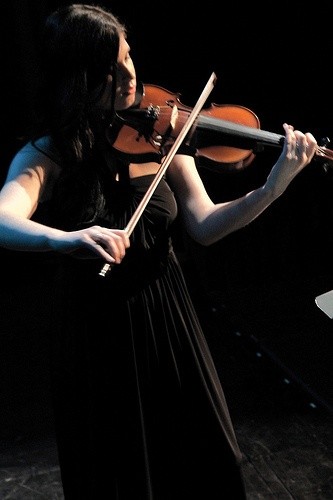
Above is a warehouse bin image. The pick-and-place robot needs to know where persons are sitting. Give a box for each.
[0,5,318,499]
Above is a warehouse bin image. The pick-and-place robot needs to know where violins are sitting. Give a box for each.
[104,82,333,170]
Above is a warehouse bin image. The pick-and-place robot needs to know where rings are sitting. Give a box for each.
[295,142,305,153]
